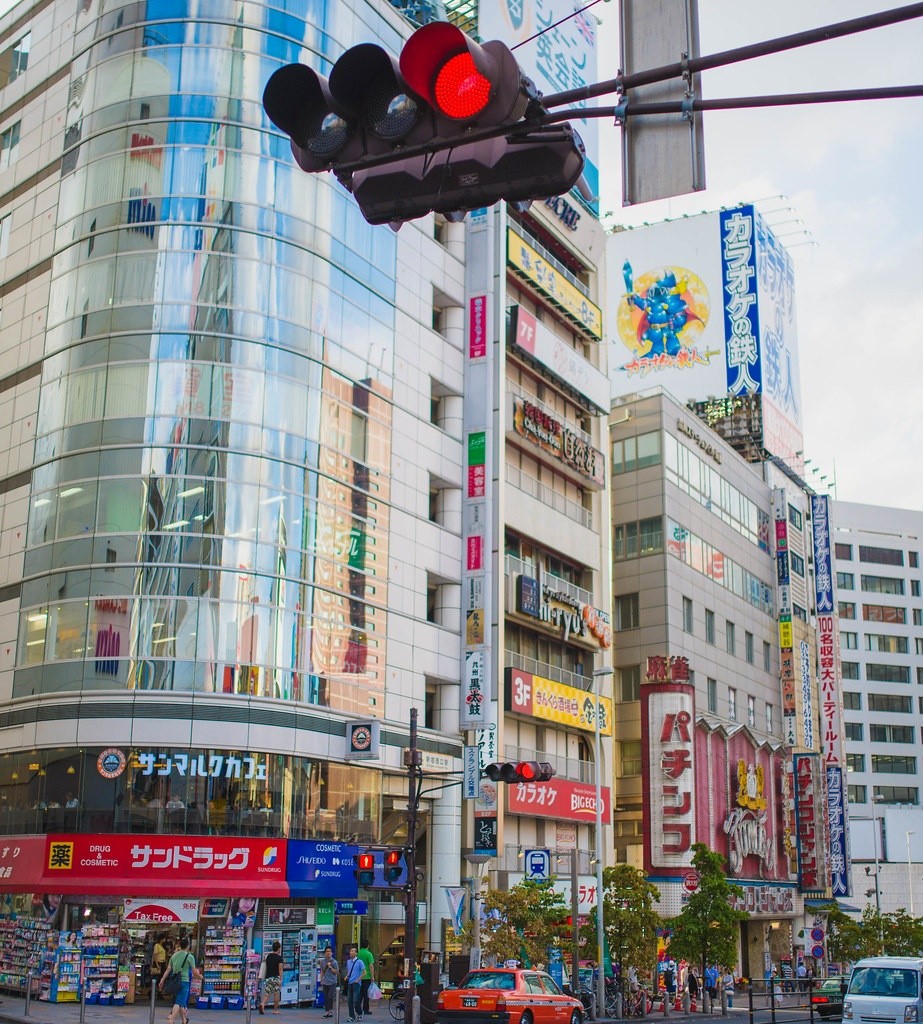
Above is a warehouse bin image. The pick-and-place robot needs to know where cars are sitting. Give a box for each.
[811,974,851,1020]
[436,965,587,1024]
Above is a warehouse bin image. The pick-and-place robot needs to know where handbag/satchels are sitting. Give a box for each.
[343,982,349,996]
[258,953,269,980]
[367,982,382,999]
[726,990,734,995]
[164,972,182,993]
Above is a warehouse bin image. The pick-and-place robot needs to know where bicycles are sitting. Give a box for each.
[388,987,406,1022]
[556,968,654,1021]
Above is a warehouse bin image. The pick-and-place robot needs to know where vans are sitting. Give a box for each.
[841,955,923,1024]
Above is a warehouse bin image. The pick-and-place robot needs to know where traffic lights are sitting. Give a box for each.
[357,852,376,886]
[263,21,535,175]
[485,760,543,784]
[383,849,403,882]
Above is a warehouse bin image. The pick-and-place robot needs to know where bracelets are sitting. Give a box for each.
[329,967,333,970]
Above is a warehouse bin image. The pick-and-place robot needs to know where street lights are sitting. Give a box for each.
[592,668,615,1019]
[871,795,885,955]
[906,831,917,927]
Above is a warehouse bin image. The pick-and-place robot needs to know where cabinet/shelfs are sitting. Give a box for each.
[130,929,174,994]
[203,925,246,995]
[0,921,119,1002]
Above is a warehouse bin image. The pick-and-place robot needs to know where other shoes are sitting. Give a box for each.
[259,1004,264,1015]
[358,1015,364,1020]
[273,1010,280,1014]
[182,1018,189,1024]
[346,1018,355,1022]
[166,1015,172,1019]
[364,1011,372,1014]
[321,1013,333,1018]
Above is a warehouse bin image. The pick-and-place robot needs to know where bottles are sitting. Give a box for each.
[57,944,119,993]
[203,925,246,990]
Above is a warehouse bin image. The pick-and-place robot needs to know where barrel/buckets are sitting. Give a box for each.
[195,995,243,1009]
[85,994,124,1005]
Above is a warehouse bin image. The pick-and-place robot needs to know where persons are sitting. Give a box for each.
[706,963,718,999]
[722,968,734,1007]
[259,941,283,1013]
[772,971,783,1007]
[357,940,374,1014]
[807,965,815,991]
[230,898,256,927]
[67,932,78,949]
[148,934,165,1000]
[686,966,699,999]
[794,962,807,996]
[320,946,338,1017]
[665,960,678,1005]
[783,967,795,996]
[344,948,366,1021]
[159,939,203,1024]
